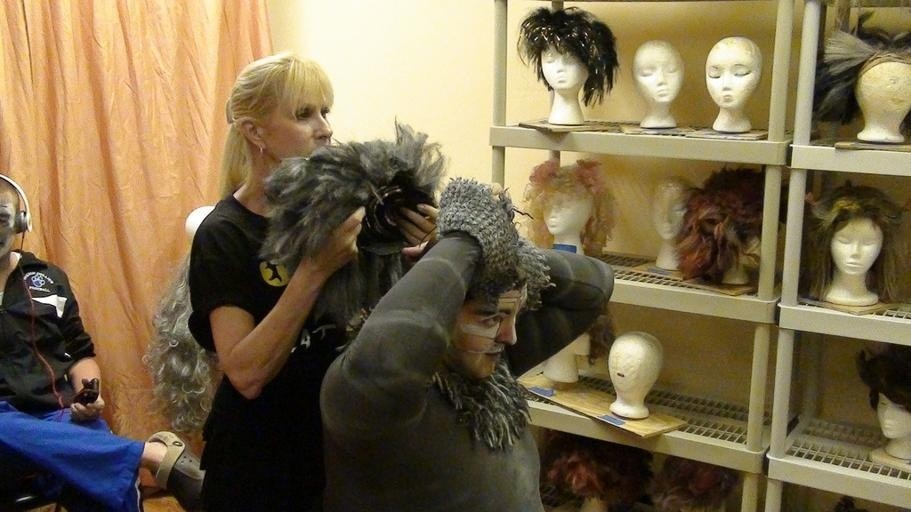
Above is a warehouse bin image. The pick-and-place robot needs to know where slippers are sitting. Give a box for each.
[146,432,206,512]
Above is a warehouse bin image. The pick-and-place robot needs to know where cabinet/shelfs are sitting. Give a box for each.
[488,1,798,510]
[763,1,911,510]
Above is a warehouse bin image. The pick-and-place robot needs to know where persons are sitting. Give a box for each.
[815,25,909,143]
[856,345,911,459]
[676,167,788,282]
[533,430,737,512]
[607,331,664,419]
[806,185,909,307]
[190,56,441,509]
[143,199,227,441]
[631,40,685,128]
[0,174,212,511]
[517,8,618,125]
[522,160,615,257]
[705,37,762,133]
[319,178,616,510]
[542,306,614,382]
[652,177,695,270]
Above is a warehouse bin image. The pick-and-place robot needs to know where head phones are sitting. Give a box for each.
[0,173,32,235]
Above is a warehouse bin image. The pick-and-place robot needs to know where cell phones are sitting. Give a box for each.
[73,379,99,405]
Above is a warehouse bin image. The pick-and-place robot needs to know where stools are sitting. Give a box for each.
[0,472,66,512]
